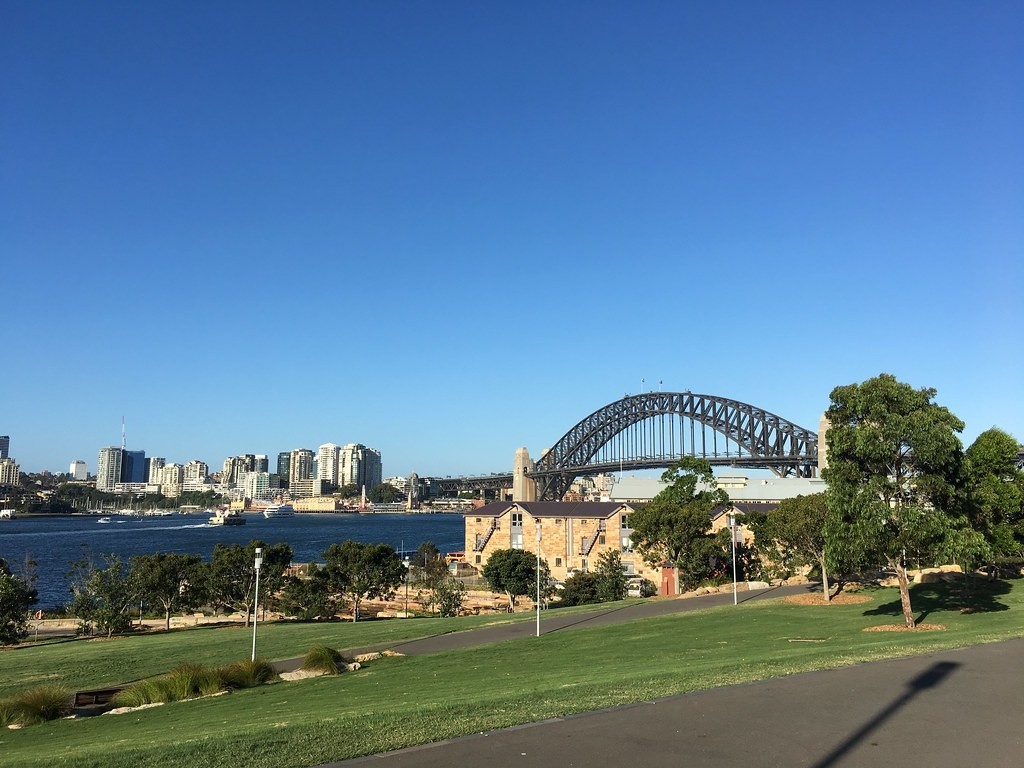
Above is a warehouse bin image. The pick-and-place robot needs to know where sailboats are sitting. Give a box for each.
[82,496,220,517]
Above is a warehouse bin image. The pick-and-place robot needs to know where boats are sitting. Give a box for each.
[262,503,295,518]
[208,505,247,526]
[95,517,113,524]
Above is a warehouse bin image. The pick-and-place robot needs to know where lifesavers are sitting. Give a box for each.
[35,611,45,620]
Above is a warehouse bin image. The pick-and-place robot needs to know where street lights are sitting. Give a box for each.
[251,547,265,664]
[729,512,738,606]
[534,525,544,638]
